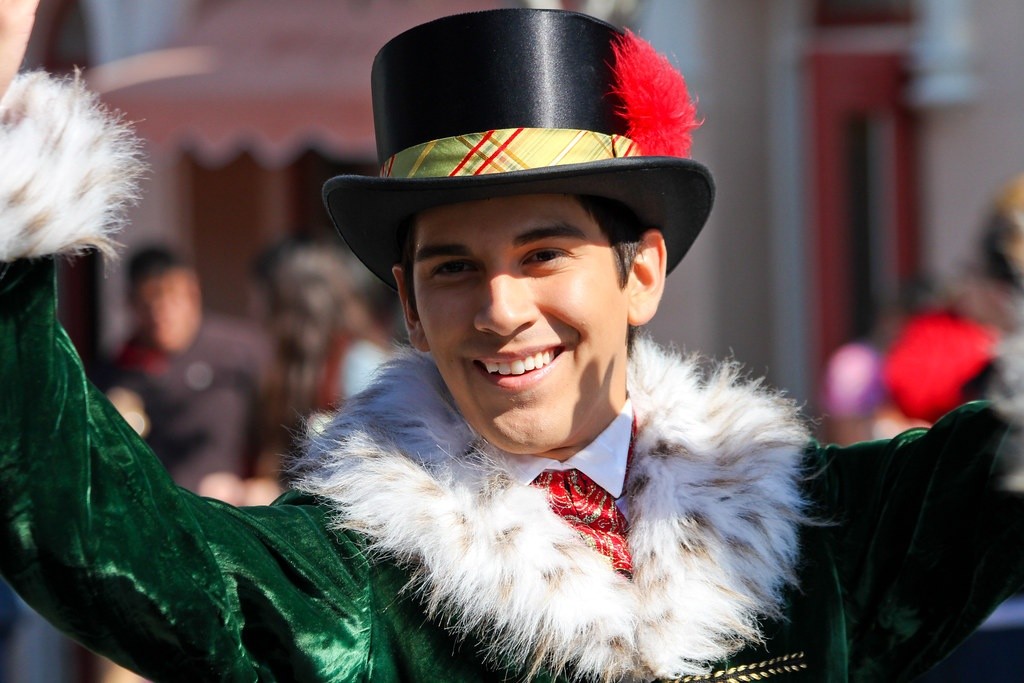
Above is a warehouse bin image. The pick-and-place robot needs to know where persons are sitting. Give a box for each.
[823,171,1024,447]
[104,238,402,507]
[0,0,1024,683]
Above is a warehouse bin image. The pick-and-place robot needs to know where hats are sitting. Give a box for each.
[322,8,717,293]
